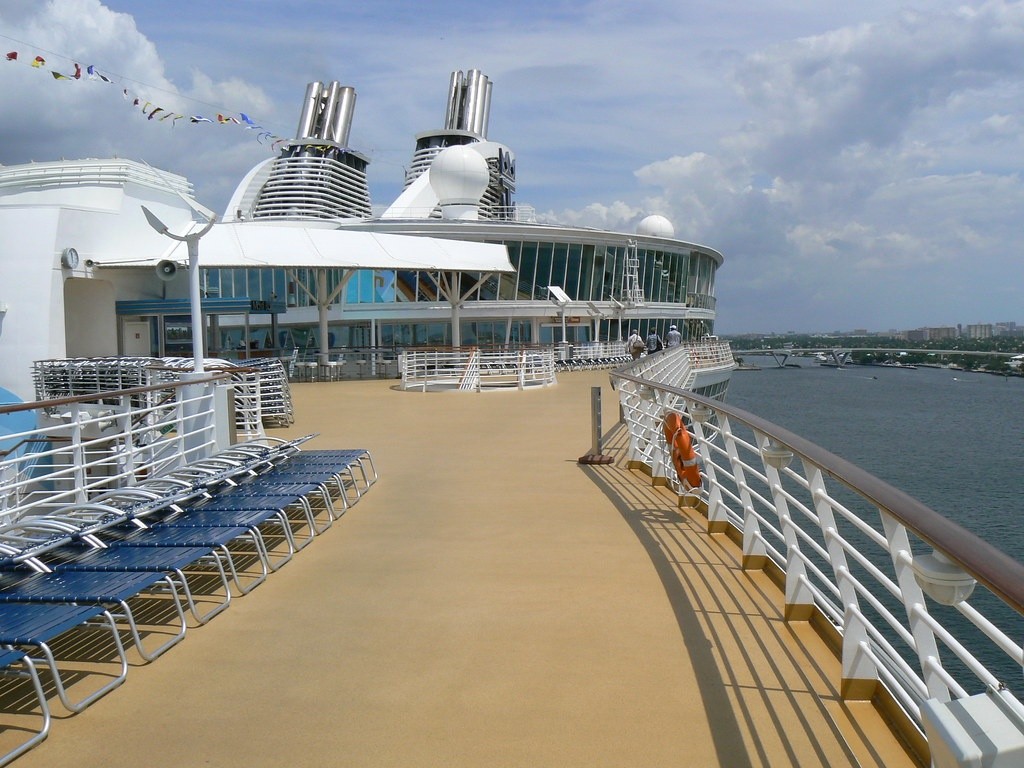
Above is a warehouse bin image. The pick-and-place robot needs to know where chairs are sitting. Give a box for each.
[0,432,378,768]
[441,356,634,377]
[29,354,294,429]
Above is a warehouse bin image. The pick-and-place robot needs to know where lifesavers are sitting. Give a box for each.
[666,412,705,491]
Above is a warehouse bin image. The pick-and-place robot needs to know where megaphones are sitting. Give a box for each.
[156,260,178,281]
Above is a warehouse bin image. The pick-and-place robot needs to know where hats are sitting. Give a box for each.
[651,327,656,331]
[670,325,676,329]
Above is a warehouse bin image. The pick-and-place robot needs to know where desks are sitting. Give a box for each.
[357,360,391,379]
[295,362,317,382]
[320,360,346,383]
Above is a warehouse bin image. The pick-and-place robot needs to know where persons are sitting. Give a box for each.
[666,325,682,348]
[646,327,663,354]
[625,329,643,360]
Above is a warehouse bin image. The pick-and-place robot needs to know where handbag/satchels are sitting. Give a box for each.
[633,335,643,348]
[657,336,662,351]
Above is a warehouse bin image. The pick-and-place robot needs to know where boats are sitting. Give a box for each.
[892,362,917,369]
[813,353,828,363]
[733,362,762,370]
